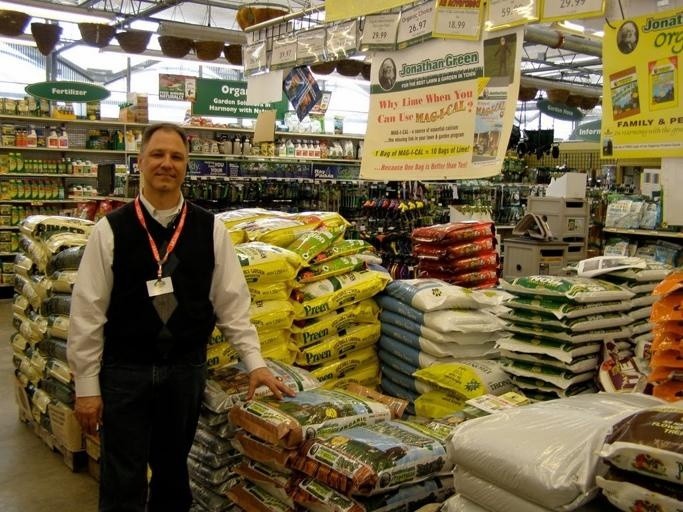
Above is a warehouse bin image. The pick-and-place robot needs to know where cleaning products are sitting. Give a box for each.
[276,138,321,158]
[26,124,38,148]
[59,125,69,149]
[46,125,60,148]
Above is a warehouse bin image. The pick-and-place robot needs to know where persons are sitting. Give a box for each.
[64,121,299,511]
[379,58,394,90]
[493,38,512,75]
[618,22,637,55]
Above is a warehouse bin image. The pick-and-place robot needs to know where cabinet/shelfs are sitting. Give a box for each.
[0,114,364,288]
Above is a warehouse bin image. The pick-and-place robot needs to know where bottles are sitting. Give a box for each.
[209,134,363,159]
[126,129,138,152]
[0,96,104,251]
